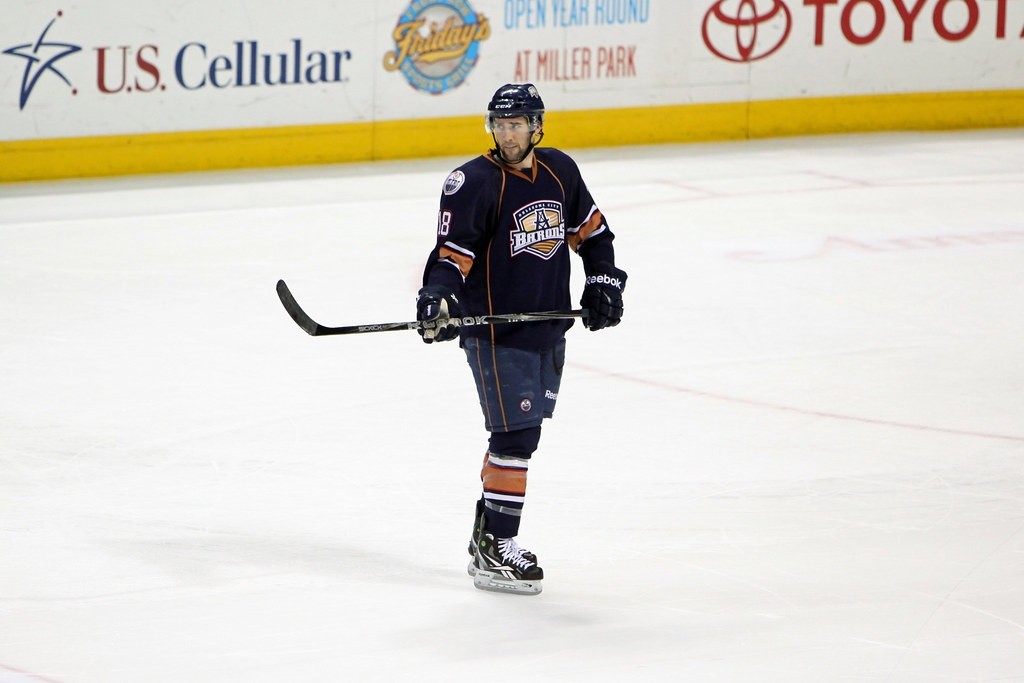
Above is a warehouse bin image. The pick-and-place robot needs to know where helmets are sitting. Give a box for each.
[485,84,546,134]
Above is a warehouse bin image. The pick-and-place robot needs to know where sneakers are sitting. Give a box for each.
[468,497,544,596]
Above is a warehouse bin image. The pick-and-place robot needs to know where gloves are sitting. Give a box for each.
[580,264,628,332]
[416,285,462,343]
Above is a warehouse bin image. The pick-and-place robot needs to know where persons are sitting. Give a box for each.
[416,83,628,595]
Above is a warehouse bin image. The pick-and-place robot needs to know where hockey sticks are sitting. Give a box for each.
[276,280,590,339]
